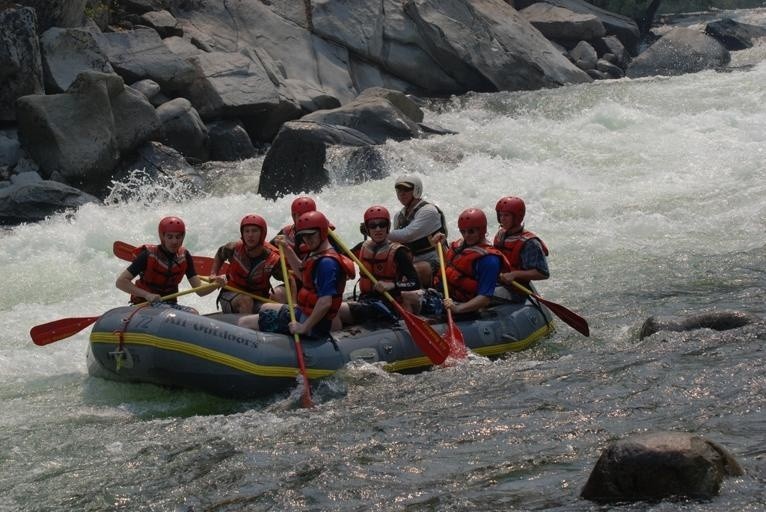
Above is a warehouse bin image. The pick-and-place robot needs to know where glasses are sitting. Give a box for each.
[460,229,476,235]
[368,221,387,228]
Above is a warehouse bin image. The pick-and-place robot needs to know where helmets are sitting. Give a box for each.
[496,197,526,225]
[295,211,329,241]
[158,216,185,248]
[395,173,422,200]
[364,206,390,234]
[292,197,316,224]
[241,215,267,246]
[458,209,487,238]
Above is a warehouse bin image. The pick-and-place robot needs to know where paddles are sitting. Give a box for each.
[30,280,223,347]
[112,240,278,304]
[278,236,312,409]
[498,273,590,338]
[191,255,231,283]
[437,239,465,360]
[327,226,450,365]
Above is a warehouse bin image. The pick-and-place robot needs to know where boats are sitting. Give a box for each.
[87,294,552,389]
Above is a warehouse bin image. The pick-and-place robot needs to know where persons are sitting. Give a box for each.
[115,217,227,316]
[362,173,447,284]
[269,197,335,280]
[495,195,550,287]
[403,208,500,321]
[210,215,282,315]
[332,207,418,330]
[237,210,355,341]
